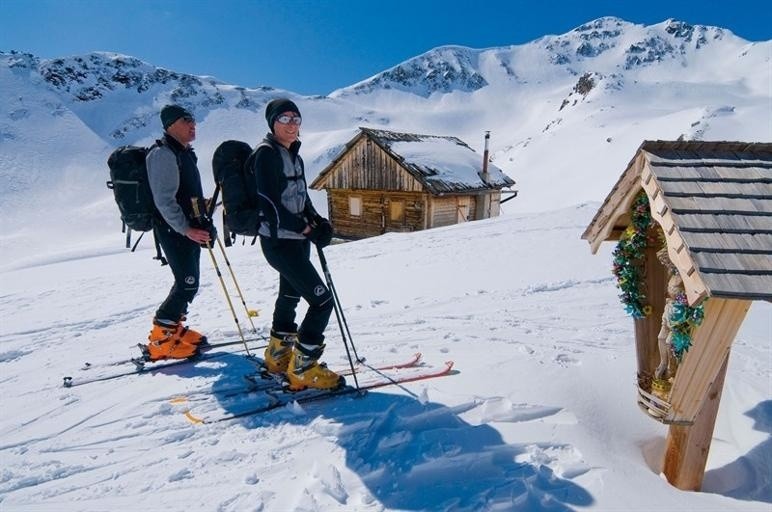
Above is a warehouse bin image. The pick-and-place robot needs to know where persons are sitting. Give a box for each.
[255,98,345,391]
[143,104,216,359]
[651,272,685,380]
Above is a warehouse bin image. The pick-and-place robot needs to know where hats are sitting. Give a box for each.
[266,99,302,135]
[161,105,185,129]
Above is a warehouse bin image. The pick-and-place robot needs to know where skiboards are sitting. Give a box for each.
[64,337,271,388]
[168,353,453,423]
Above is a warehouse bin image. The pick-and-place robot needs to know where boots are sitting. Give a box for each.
[287,339,345,389]
[174,322,206,345]
[149,316,199,359]
[264,329,296,373]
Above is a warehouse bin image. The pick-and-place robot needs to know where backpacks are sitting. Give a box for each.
[211,140,283,247]
[107,139,185,267]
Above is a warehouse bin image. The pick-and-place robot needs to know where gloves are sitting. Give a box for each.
[303,218,334,247]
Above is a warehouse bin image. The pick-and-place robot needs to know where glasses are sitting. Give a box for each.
[183,114,196,123]
[277,115,302,125]
